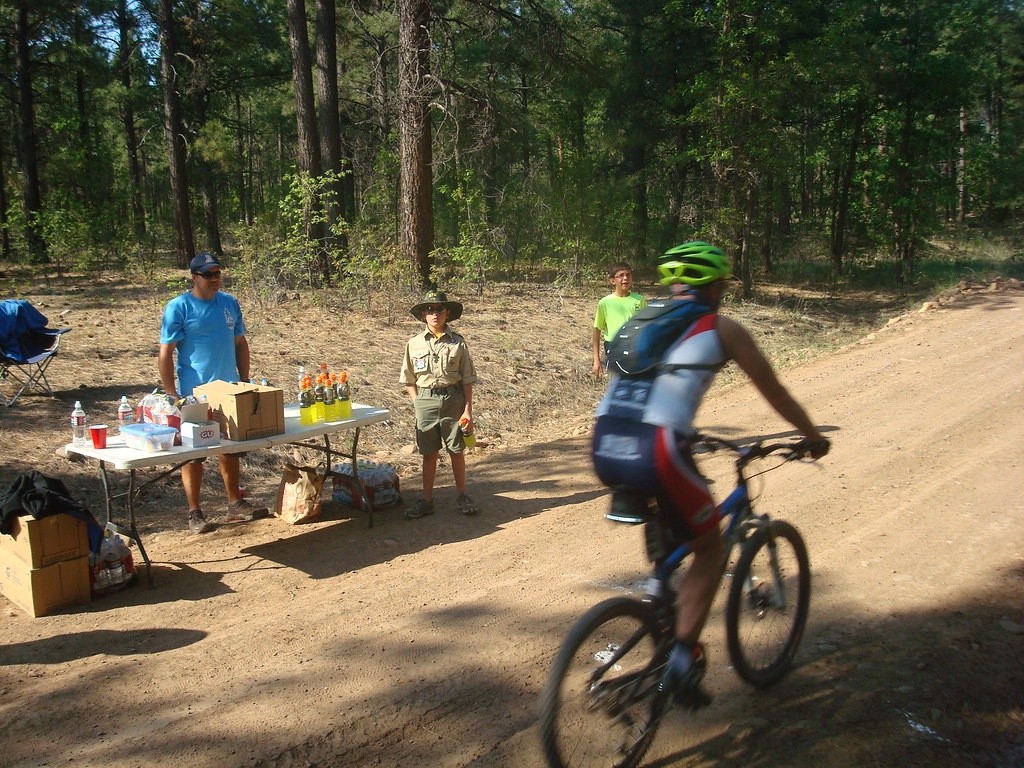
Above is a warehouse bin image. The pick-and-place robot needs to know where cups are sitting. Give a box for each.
[89,425,108,449]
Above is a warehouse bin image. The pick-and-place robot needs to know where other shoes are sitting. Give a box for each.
[404,499,436,518]
[661,642,715,711]
[456,493,479,514]
[639,590,680,613]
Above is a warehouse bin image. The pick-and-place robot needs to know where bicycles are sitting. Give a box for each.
[538,434,813,768]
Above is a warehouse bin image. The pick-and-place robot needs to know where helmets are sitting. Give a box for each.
[657,240,733,294]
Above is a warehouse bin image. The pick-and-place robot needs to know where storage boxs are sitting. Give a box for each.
[0,510,90,569]
[0,545,91,618]
[180,403,220,448]
[117,423,179,452]
[192,379,285,441]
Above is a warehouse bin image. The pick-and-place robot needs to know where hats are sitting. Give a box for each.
[409,289,463,322]
[190,253,222,274]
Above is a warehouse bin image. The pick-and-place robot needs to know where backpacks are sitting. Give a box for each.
[607,299,723,378]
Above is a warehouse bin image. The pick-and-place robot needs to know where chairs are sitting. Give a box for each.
[0,299,72,406]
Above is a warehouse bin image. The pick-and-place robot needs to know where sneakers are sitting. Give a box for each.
[188,510,209,535]
[226,500,269,522]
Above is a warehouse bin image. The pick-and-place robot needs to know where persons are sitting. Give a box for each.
[592,242,831,708]
[158,252,269,535]
[398,289,479,519]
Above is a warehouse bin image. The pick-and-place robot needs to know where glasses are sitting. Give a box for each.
[195,271,222,279]
[425,305,446,313]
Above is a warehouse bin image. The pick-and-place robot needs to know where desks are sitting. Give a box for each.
[64,401,390,595]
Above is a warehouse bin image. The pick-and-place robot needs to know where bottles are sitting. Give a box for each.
[262,378,267,385]
[460,417,476,447]
[88,529,137,590]
[299,366,305,390]
[314,372,336,423]
[250,379,255,384]
[229,380,233,383]
[200,395,212,421]
[329,372,352,421]
[333,459,399,510]
[319,362,329,380]
[71,401,86,448]
[118,396,134,440]
[298,375,317,426]
